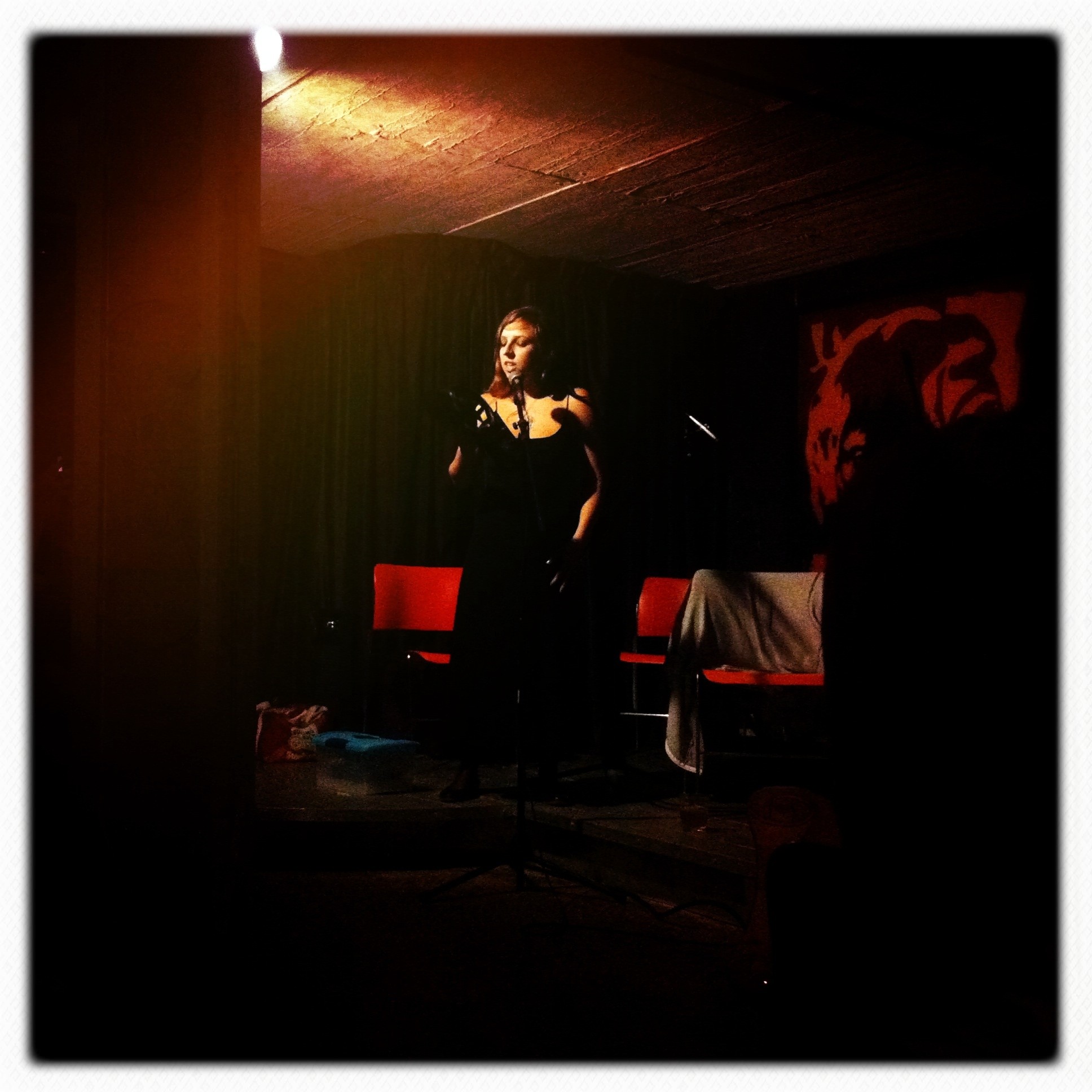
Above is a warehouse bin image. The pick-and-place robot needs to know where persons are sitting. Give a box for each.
[445,307,620,798]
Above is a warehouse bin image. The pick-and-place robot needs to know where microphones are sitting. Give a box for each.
[513,377,525,405]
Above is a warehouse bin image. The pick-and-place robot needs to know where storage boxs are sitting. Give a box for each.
[312,729,421,795]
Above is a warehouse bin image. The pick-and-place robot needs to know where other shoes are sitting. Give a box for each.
[439,768,479,802]
[541,765,565,805]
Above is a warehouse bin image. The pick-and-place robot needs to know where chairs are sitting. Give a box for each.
[352,566,835,765]
